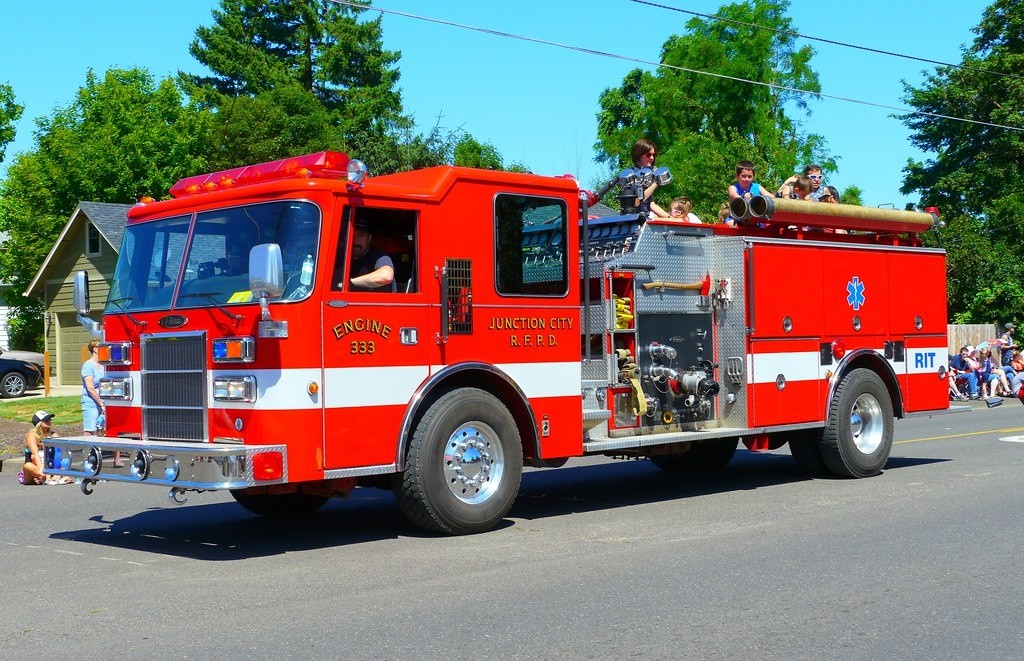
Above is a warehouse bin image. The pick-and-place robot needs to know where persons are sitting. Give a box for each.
[337,218,397,293]
[619,139,675,219]
[674,195,703,224]
[80,339,125,472]
[655,201,690,222]
[775,164,854,235]
[18,410,73,484]
[724,161,775,228]
[999,323,1019,367]
[948,347,1024,402]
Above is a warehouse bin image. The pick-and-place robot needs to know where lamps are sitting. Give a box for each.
[45,310,51,325]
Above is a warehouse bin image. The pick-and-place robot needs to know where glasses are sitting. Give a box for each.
[645,153,656,158]
[806,173,822,180]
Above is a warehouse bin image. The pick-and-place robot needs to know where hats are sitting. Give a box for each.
[32,410,55,426]
[948,353,954,361]
[1005,323,1016,329]
[344,217,377,234]
[967,345,979,356]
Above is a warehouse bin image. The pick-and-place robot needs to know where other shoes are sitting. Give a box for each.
[970,395,979,399]
[997,391,1013,397]
[114,461,125,468]
[957,394,968,401]
[979,396,990,401]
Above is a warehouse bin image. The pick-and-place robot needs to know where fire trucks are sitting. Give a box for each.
[41,149,947,537]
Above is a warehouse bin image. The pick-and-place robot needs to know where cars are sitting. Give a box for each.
[0,344,44,398]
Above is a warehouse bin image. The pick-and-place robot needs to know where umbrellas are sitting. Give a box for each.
[975,339,1008,351]
